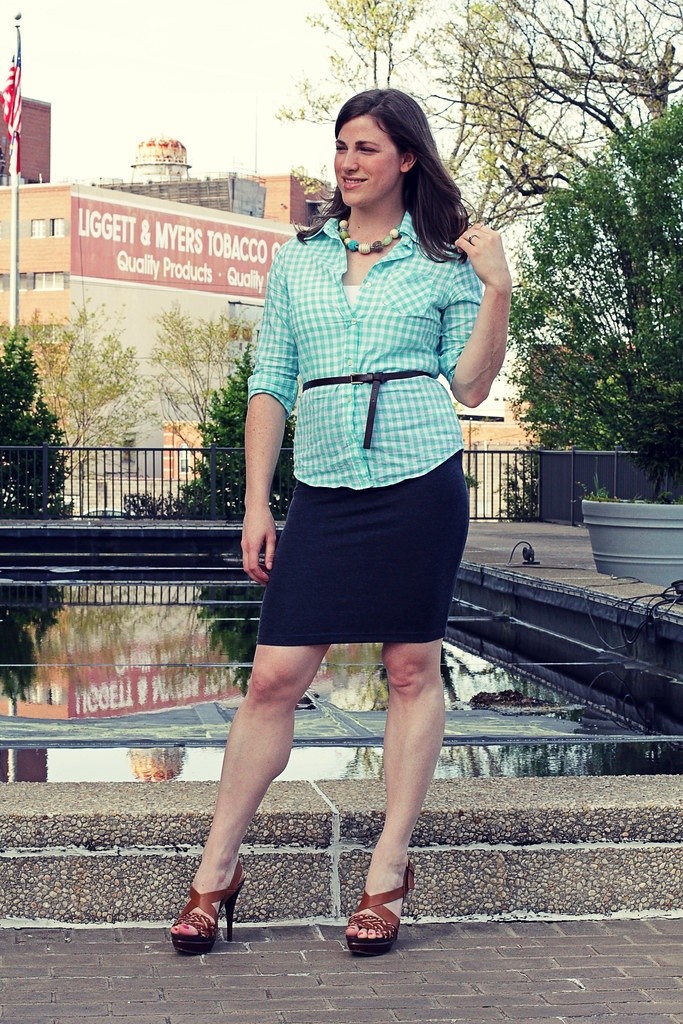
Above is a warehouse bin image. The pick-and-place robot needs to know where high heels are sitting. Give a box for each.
[171,859,246,953]
[346,859,415,955]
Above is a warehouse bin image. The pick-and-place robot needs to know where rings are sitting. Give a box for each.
[469,234,478,245]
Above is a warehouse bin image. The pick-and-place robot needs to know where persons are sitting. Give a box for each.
[172,89,511,955]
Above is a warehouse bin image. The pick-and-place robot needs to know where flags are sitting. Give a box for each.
[0,39,22,173]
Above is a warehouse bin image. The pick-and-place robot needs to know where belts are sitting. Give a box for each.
[303,370,432,449]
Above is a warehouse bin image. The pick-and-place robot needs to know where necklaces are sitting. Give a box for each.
[339,220,400,254]
